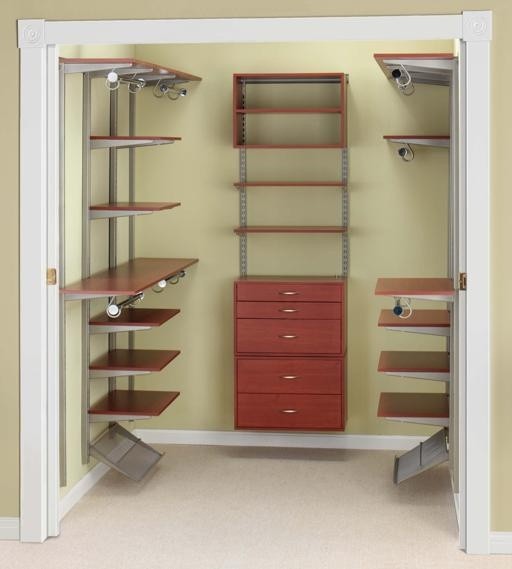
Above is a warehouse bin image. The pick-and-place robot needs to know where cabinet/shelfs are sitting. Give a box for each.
[230,73,350,277]
[58,56,202,487]
[232,280,348,432]
[372,51,458,486]
